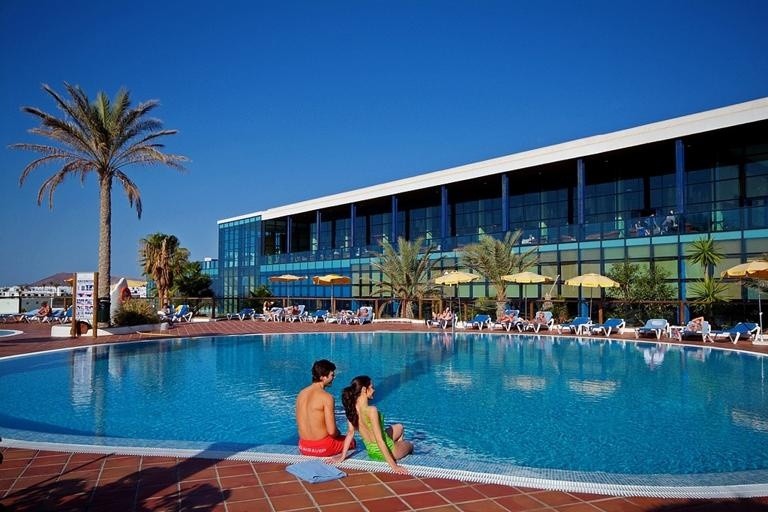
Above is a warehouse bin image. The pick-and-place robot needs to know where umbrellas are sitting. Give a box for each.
[437,362,477,394]
[500,366,548,395]
[269,273,309,304]
[498,270,554,317]
[564,368,622,400]
[310,272,351,306]
[717,258,768,335]
[564,271,621,319]
[433,272,481,317]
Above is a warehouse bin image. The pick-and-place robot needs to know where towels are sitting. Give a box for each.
[285,457,346,485]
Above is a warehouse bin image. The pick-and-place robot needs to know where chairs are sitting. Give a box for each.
[0,304,75,324]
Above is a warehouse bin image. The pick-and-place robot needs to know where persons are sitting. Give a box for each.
[326,373,417,478]
[634,209,679,236]
[292,358,358,457]
[529,313,547,325]
[262,300,370,324]
[500,312,516,322]
[688,315,704,333]
[159,302,177,327]
[432,306,454,321]
[35,299,52,323]
